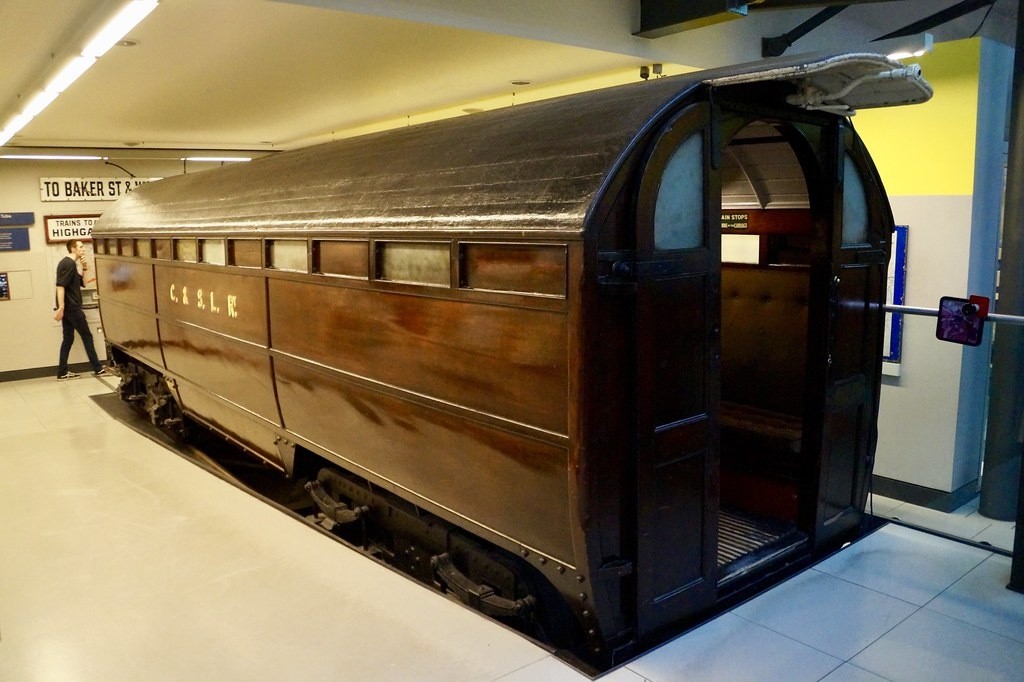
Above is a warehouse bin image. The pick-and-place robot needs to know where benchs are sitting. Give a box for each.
[716,397,804,450]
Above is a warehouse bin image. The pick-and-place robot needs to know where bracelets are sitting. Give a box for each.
[83,268,88,271]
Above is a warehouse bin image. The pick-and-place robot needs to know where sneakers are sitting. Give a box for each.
[57,371,81,380]
[95,366,116,377]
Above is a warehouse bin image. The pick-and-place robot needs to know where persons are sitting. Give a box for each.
[52,239,114,381]
